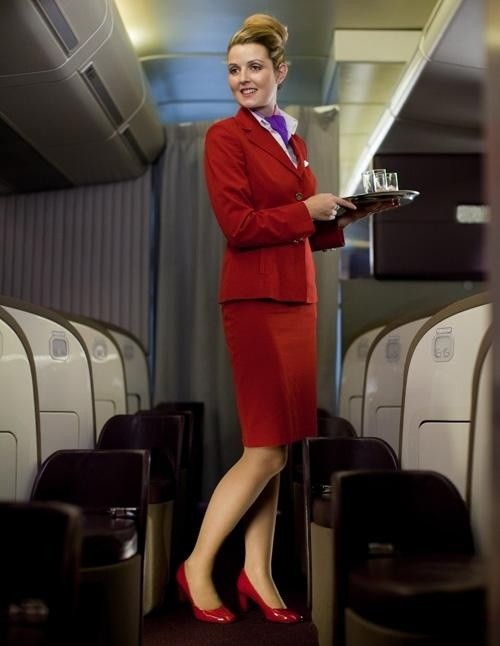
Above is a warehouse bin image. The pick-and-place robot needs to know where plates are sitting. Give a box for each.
[339,190,420,208]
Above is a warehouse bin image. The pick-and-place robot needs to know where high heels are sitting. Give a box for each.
[176,561,236,623]
[238,569,305,624]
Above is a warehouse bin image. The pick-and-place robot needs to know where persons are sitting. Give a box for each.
[175,14,399,624]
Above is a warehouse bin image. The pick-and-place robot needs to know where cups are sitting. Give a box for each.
[362,169,398,193]
